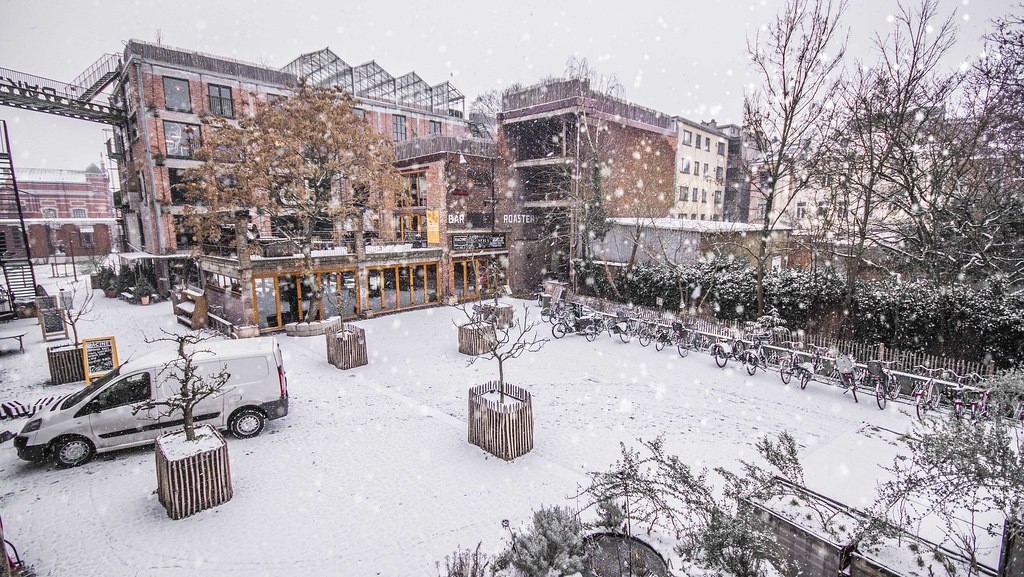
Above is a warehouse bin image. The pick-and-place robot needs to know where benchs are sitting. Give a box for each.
[121,292,134,304]
[0,330,29,354]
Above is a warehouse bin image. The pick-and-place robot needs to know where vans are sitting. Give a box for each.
[13,333,289,469]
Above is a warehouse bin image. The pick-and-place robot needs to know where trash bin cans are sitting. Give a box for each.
[541,294,551,315]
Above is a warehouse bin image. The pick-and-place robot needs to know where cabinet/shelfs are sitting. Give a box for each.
[175,285,208,331]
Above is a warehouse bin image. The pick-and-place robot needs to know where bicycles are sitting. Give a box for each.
[742,333,768,376]
[534,285,710,358]
[944,368,996,422]
[868,360,903,411]
[800,344,830,390]
[713,327,746,368]
[912,365,946,421]
[834,354,859,402]
[778,341,801,379]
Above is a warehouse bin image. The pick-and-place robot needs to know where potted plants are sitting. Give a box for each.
[106,279,119,298]
[140,289,150,305]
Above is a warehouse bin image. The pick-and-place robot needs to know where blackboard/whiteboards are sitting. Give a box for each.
[34,296,58,317]
[36,306,68,337]
[82,337,118,379]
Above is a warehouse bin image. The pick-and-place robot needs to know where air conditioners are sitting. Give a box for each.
[114,190,129,209]
[105,137,123,157]
